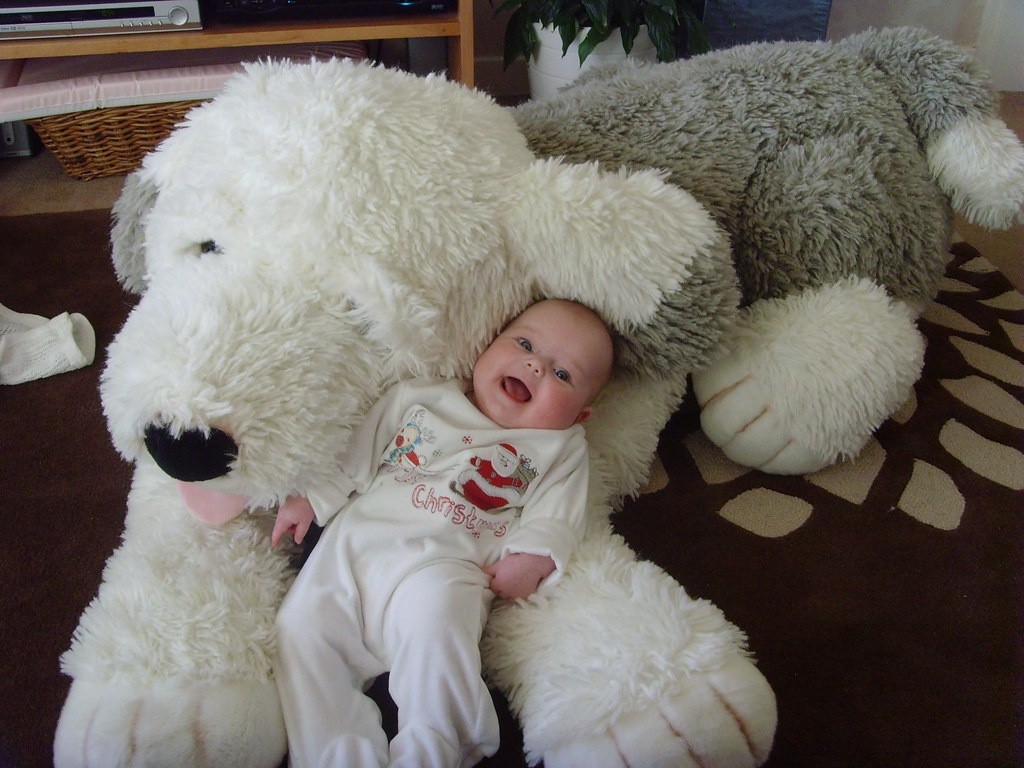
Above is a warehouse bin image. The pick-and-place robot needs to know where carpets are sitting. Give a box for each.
[0,207,1024,768]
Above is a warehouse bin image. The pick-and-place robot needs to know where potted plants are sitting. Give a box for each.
[493,0,737,102]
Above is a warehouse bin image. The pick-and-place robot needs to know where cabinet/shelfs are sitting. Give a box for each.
[0,0,475,216]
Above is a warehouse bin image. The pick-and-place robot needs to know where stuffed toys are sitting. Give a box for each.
[54,26,1024,768]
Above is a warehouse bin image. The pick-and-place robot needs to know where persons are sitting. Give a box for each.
[270,301,614,768]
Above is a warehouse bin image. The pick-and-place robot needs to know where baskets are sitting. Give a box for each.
[22,98,214,183]
